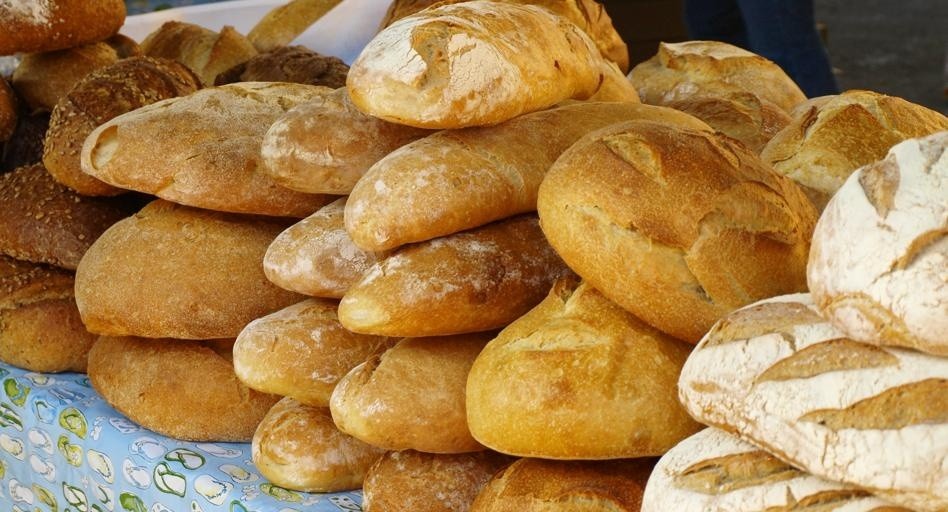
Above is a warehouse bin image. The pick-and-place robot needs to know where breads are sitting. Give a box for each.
[198,24,258,88]
[12,43,118,109]
[371,2,629,74]
[264,196,397,301]
[475,458,644,512]
[217,46,350,87]
[2,259,87,375]
[80,80,341,226]
[246,0,344,47]
[81,337,278,441]
[538,117,821,345]
[767,91,948,199]
[809,129,948,356]
[341,101,719,255]
[258,84,430,197]
[333,337,504,456]
[362,452,497,511]
[41,53,198,199]
[345,1,606,131]
[470,286,697,460]
[678,287,948,511]
[142,21,218,74]
[334,213,565,338]
[638,427,898,510]
[243,396,380,493]
[2,0,129,57]
[234,300,404,401]
[0,164,129,272]
[72,199,303,341]
[629,36,808,174]
